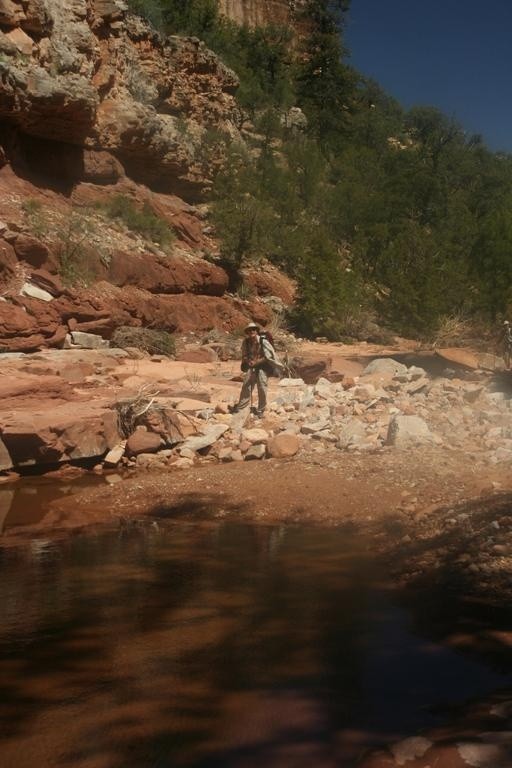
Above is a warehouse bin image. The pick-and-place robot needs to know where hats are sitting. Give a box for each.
[244,322,260,334]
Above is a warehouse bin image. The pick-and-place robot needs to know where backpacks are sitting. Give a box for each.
[259,331,276,353]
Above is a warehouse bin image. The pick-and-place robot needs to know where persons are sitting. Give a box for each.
[227,322,283,415]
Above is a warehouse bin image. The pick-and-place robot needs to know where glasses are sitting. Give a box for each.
[248,329,256,332]
